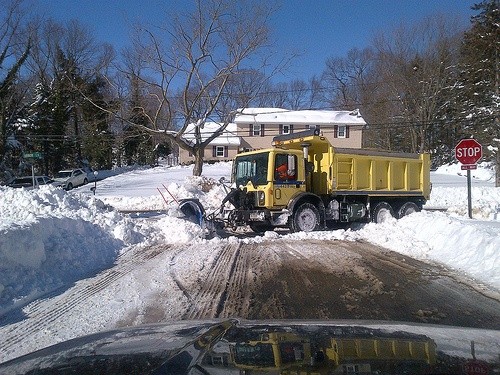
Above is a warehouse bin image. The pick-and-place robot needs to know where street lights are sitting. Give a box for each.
[92,171,97,190]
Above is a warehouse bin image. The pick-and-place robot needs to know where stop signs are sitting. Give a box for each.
[455,139,481,165]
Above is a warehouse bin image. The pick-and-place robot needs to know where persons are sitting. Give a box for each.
[274,158,294,180]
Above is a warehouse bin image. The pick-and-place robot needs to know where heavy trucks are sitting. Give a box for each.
[214,129,431,235]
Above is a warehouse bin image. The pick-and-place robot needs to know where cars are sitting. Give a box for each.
[52,168,88,191]
[9,175,62,193]
